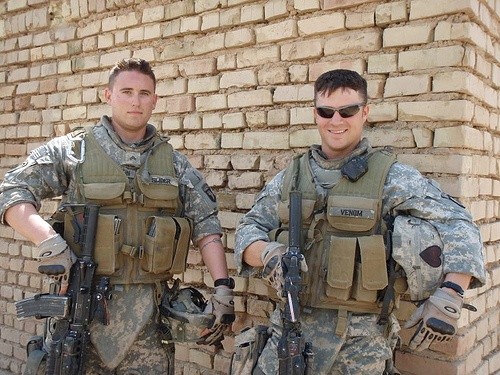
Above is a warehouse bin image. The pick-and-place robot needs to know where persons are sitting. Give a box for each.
[0,58,235,375]
[234,69,486,375]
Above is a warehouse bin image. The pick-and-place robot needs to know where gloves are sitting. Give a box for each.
[194,286,239,346]
[402,288,463,351]
[260,242,309,293]
[31,235,77,297]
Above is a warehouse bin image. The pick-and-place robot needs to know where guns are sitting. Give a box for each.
[274,192,316,375]
[15,203,100,375]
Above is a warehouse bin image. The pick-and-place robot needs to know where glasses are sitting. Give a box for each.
[315,101,367,119]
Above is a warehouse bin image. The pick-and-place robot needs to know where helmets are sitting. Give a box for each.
[393,216,445,302]
[161,288,207,344]
